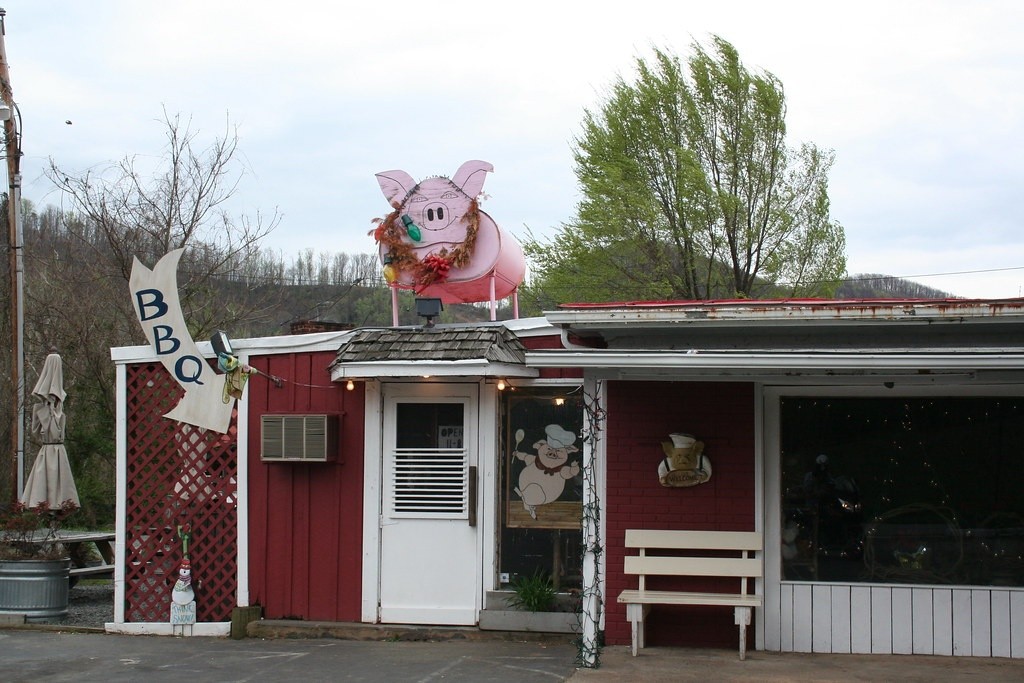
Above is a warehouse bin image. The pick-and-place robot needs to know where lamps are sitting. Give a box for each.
[347,377,354,390]
[415,298,444,328]
[210,330,233,357]
[498,376,506,390]
[552,394,566,405]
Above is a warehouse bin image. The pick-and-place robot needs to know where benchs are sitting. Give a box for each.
[69,562,151,576]
[618,529,763,661]
[132,546,170,556]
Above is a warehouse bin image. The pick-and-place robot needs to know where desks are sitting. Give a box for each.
[0,527,116,566]
[137,527,172,561]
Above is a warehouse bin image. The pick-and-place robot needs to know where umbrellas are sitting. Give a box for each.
[20,346,82,556]
[174,422,210,512]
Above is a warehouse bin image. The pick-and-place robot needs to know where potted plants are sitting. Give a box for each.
[0,500,85,624]
